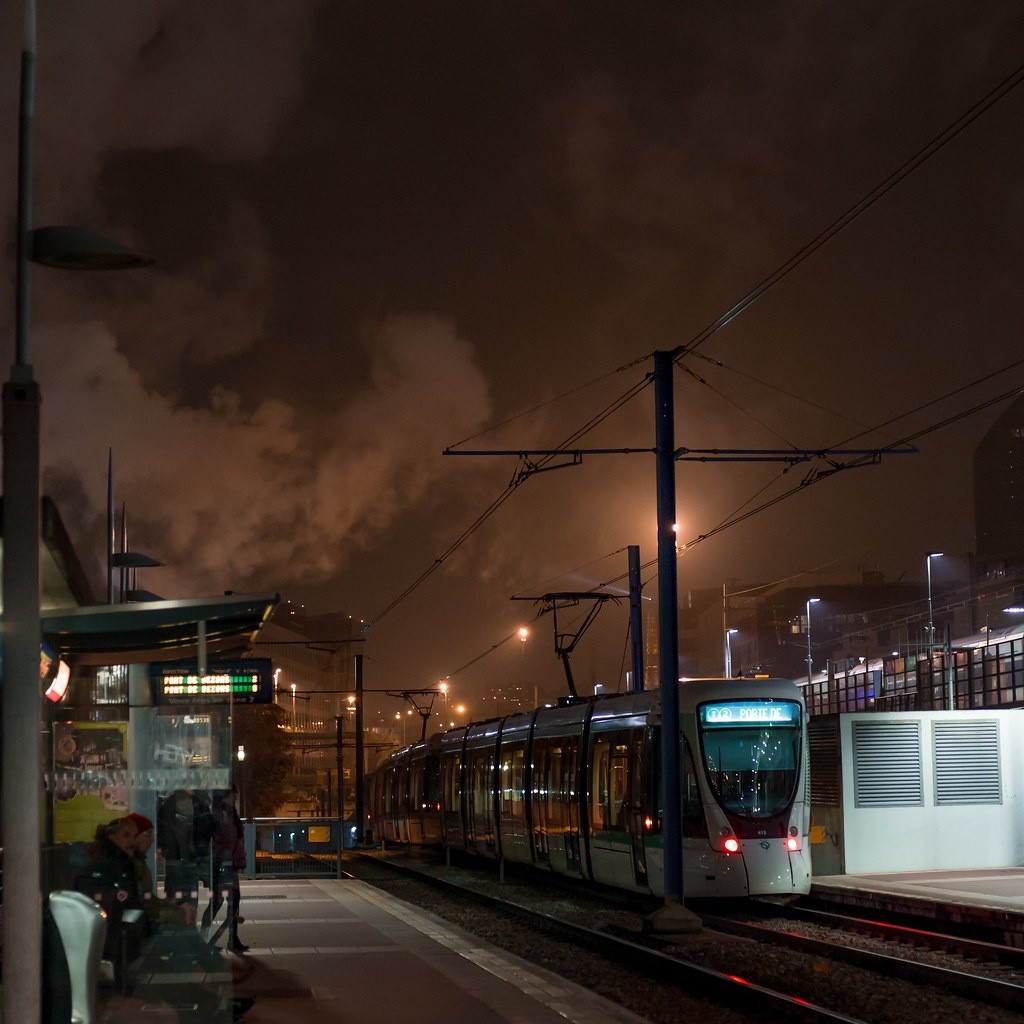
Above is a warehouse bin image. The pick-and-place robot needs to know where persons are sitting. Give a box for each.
[157,765,250,953]
[68,813,257,1024]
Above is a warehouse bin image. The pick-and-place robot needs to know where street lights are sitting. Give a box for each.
[806,599,821,682]
[928,553,944,651]
[726,629,739,679]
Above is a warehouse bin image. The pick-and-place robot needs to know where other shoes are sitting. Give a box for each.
[227,935,248,951]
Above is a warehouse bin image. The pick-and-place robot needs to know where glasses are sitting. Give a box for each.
[142,831,152,837]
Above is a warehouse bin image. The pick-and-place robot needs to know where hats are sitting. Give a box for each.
[128,813,154,839]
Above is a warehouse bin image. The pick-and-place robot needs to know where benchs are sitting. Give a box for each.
[68,879,145,994]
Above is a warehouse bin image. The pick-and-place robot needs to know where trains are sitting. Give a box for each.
[365,591,809,909]
